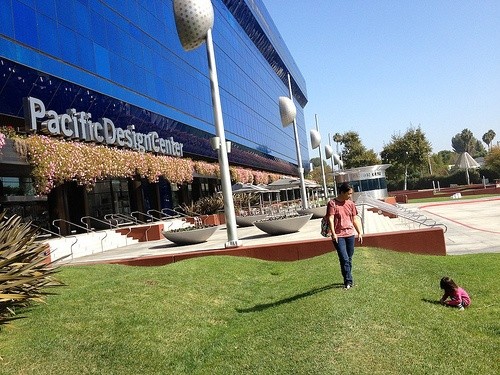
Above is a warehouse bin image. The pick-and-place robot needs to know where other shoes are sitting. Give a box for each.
[343,284,350,290]
[456,303,464,311]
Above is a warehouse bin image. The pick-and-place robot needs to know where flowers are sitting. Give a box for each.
[0,124,299,199]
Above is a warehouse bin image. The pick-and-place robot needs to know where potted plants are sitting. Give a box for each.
[162,224,218,244]
[253,212,314,235]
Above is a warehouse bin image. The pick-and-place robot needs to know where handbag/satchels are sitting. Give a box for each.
[321,214,338,237]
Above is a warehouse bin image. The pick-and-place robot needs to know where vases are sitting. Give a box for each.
[0,138,233,180]
[298,205,326,218]
[236,214,267,226]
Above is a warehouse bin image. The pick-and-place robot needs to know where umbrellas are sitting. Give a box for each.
[455,152,480,185]
[217,176,322,209]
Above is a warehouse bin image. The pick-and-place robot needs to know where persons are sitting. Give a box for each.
[327,184,363,290]
[440,276,471,311]
